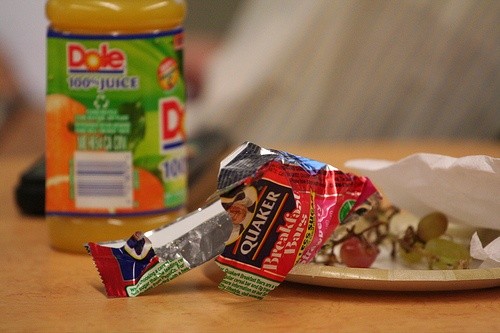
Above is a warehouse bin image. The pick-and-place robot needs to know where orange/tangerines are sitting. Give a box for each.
[46,94,162,214]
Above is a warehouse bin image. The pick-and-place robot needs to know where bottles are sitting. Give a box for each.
[42,0,191,257]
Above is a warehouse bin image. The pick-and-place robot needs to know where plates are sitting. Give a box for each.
[214,229,500,292]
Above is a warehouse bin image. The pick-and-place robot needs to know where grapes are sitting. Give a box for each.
[338,210,500,269]
